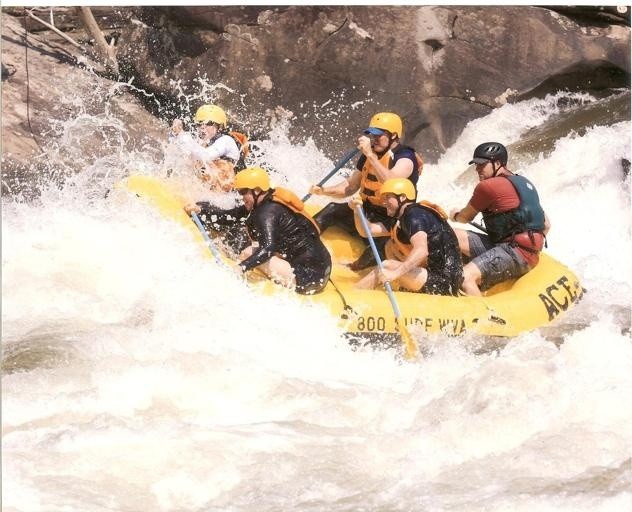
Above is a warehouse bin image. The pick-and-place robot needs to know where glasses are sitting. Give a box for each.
[197,121,215,126]
[238,189,249,195]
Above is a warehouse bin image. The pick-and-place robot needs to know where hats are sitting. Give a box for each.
[364,127,384,136]
[469,157,490,165]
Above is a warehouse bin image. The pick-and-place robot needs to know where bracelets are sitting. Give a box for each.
[453,212,460,222]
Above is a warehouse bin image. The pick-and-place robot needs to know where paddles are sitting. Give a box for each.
[351,198,419,358]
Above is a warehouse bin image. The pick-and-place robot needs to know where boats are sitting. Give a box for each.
[104,174,586,356]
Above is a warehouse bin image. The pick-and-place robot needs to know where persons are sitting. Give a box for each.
[308,112,423,273]
[352,177,462,296]
[447,142,551,298]
[183,167,332,295]
[172,105,248,210]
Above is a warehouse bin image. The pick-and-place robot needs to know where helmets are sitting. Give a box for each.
[234,168,270,191]
[473,143,507,165]
[380,178,416,201]
[195,104,227,130]
[369,112,403,139]
